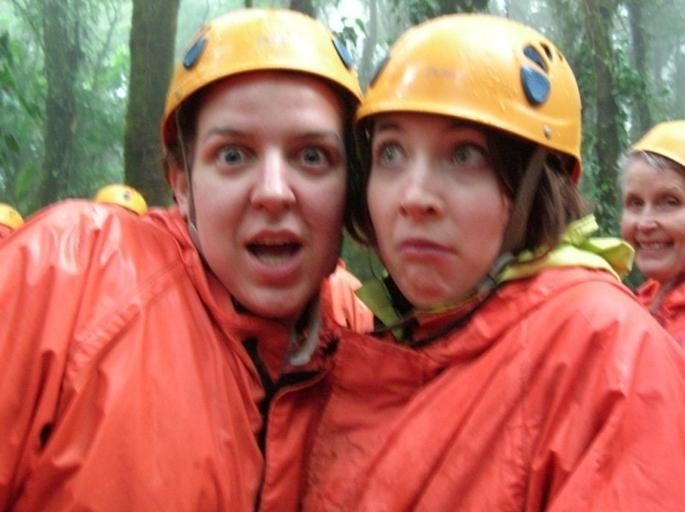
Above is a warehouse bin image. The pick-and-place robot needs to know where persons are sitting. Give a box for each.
[0,7,371,511]
[254,11,684,510]
[605,115,684,346]
[0,199,29,245]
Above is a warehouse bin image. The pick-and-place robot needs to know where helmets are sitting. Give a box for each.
[354,12,585,182]
[159,5,364,176]
[630,119,685,171]
[0,201,28,232]
[89,181,149,218]
